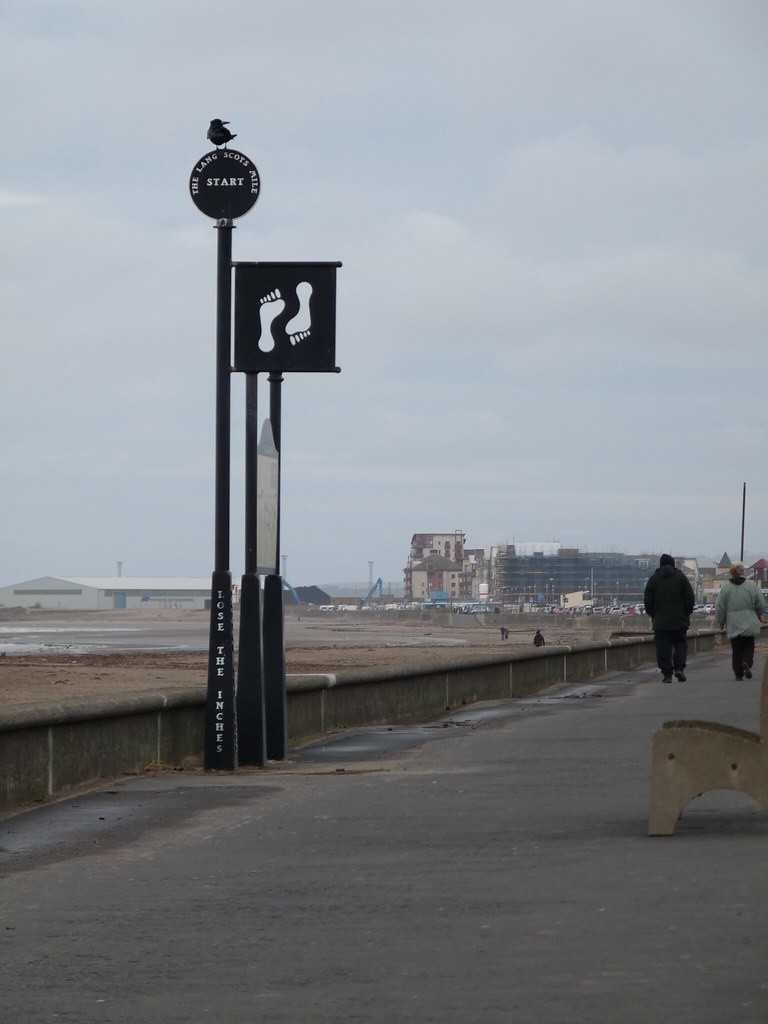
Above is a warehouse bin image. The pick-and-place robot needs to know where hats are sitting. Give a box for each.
[536,631,540,633]
[660,554,675,567]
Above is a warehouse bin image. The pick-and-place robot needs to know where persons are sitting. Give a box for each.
[501,625,509,641]
[640,607,643,614]
[715,563,768,681]
[644,553,696,683]
[534,630,545,647]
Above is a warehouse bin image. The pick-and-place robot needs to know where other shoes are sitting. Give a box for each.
[735,676,743,681]
[742,661,752,679]
[674,669,686,682]
[663,674,672,683]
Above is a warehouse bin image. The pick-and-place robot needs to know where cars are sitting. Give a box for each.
[455,603,491,615]
[692,603,717,615]
[544,603,648,615]
